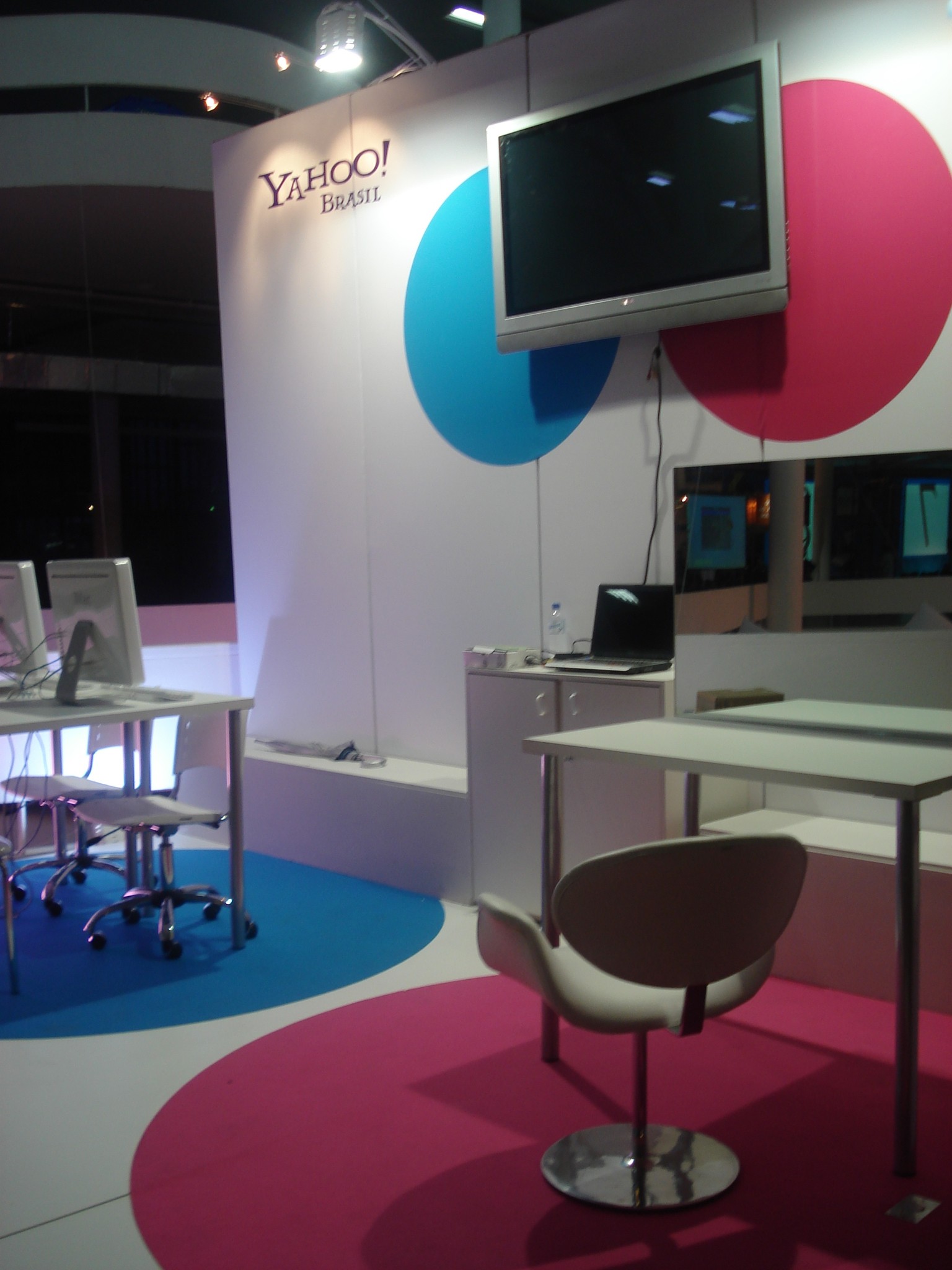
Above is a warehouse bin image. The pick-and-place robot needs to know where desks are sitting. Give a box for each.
[0,672,257,949]
[523,698,952,1179]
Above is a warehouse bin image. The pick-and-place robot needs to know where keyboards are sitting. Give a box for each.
[101,683,194,702]
[17,669,92,692]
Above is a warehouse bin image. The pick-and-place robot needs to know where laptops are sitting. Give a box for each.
[545,585,676,674]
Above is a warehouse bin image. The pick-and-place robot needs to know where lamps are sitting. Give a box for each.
[312,1,436,76]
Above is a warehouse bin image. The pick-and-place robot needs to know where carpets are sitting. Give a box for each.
[130,974,952,1270]
[0,849,445,1039]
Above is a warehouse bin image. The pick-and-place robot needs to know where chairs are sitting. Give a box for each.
[73,711,233,959]
[0,720,159,917]
[0,836,21,995]
[473,833,808,1212]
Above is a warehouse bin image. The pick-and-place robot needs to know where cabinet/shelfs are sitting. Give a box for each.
[467,668,673,917]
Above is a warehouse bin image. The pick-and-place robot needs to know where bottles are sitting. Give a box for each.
[549,603,566,653]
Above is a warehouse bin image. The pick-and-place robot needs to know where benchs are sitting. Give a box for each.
[698,807,952,1013]
[220,736,478,905]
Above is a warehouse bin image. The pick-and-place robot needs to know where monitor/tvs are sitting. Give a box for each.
[48,557,146,706]
[0,560,48,695]
[487,40,790,353]
